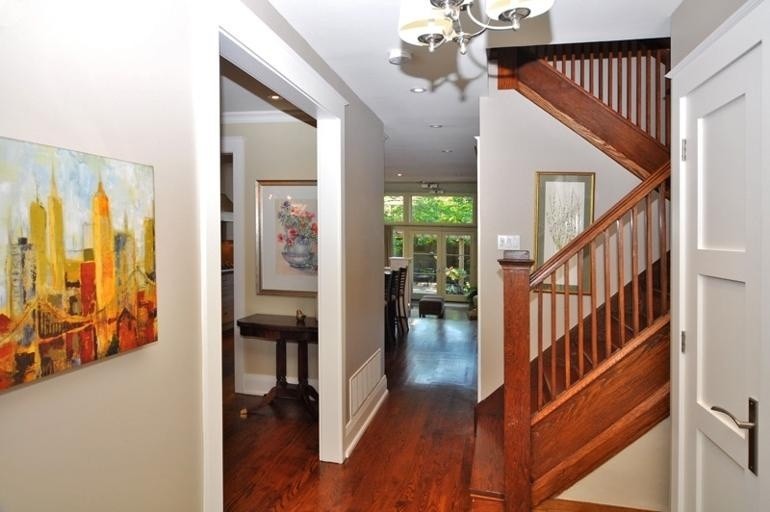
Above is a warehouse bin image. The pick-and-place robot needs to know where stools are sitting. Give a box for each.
[418,295,445,319]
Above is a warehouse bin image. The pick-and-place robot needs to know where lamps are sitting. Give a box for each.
[398,0,555,53]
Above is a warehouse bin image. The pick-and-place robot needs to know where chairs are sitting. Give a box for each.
[384,265,410,342]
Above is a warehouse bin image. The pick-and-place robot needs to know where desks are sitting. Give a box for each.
[237,313,318,423]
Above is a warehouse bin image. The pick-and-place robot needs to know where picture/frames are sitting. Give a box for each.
[532,170,596,295]
[253,178,317,297]
